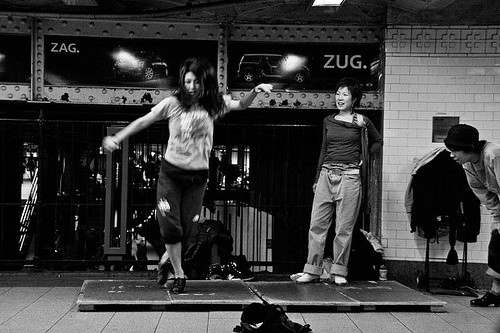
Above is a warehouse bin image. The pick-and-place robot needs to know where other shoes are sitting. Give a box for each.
[206,264,222,280]
[296,273,320,283]
[335,275,348,286]
[222,263,254,281]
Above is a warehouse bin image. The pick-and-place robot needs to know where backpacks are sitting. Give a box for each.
[182,219,232,280]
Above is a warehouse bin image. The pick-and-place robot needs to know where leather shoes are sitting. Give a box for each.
[471,291,500,307]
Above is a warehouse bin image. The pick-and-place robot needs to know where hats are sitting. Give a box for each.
[443,123,480,149]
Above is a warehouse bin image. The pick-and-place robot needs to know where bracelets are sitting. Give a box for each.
[253,87,257,94]
[113,136,118,145]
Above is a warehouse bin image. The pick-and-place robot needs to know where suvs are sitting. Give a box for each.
[237,54,310,83]
[113,50,168,81]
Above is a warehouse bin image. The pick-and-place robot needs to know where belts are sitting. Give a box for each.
[320,168,360,175]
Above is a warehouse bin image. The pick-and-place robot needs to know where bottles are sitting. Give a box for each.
[379,259,388,281]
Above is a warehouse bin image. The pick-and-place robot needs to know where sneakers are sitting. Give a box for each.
[157,261,170,286]
[171,277,186,294]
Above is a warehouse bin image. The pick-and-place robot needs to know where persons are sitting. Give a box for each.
[444,124,500,307]
[103,59,273,292]
[297,77,384,287]
